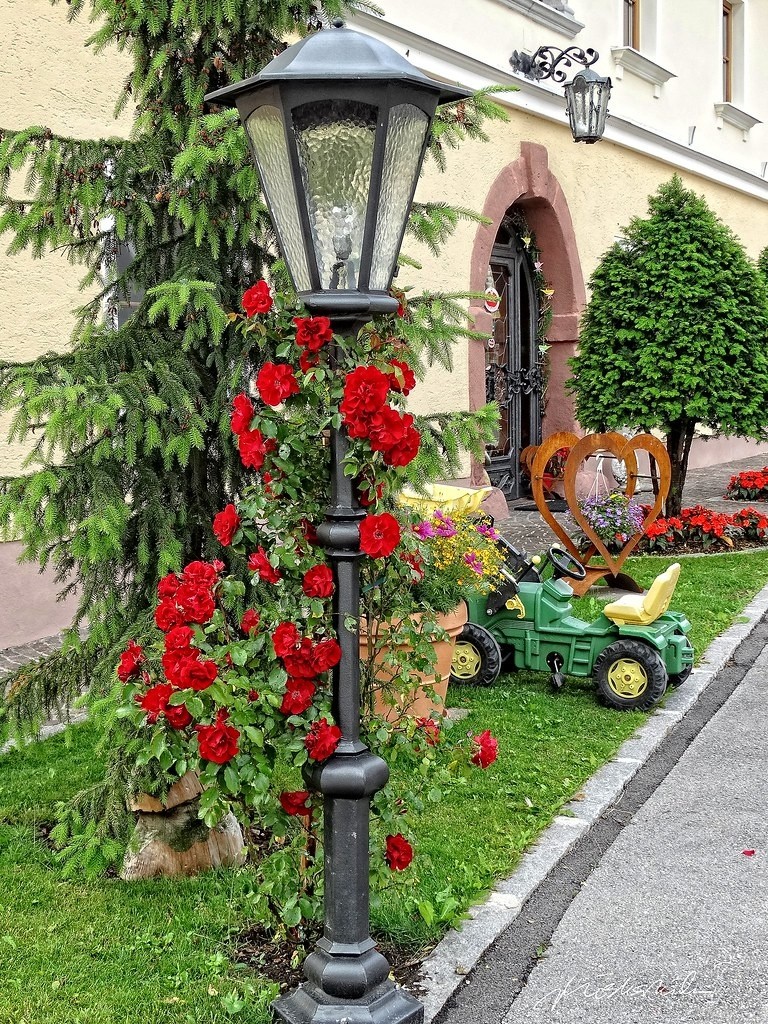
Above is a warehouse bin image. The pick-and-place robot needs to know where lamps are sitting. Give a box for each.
[509,46,613,145]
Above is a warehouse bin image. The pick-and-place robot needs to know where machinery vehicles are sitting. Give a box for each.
[450,514,695,713]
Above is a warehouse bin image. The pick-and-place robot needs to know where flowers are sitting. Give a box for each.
[566,492,644,549]
[399,494,510,615]
[544,447,570,477]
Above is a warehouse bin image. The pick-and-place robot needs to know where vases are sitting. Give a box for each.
[361,596,468,729]
[542,473,555,490]
[581,538,612,554]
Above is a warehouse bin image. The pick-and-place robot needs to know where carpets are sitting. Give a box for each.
[516,499,570,512]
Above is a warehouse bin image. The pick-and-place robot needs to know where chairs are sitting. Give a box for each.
[603,562,681,629]
